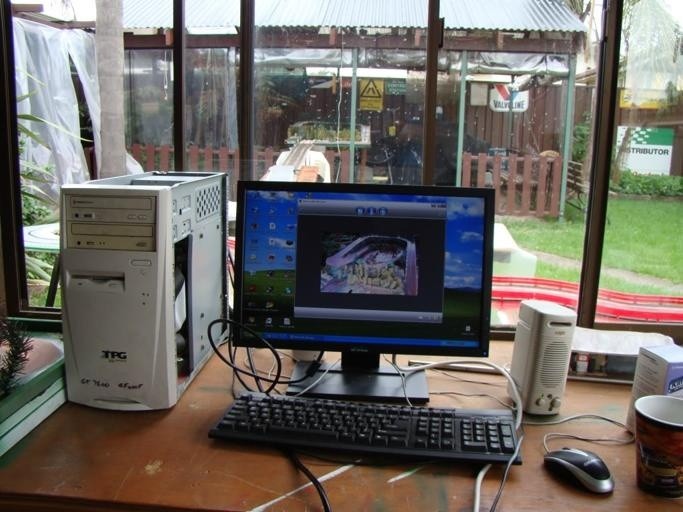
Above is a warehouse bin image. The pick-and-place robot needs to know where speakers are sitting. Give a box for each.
[508,299,578,417]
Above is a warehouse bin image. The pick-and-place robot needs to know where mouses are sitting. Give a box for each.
[543,447,615,495]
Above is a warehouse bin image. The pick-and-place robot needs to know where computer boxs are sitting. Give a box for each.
[60,170,230,411]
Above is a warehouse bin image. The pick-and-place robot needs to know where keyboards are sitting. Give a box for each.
[207,390,523,468]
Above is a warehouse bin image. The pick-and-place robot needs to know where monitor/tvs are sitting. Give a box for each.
[232,182,496,397]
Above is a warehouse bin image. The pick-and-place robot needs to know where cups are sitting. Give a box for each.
[633,395,683,500]
